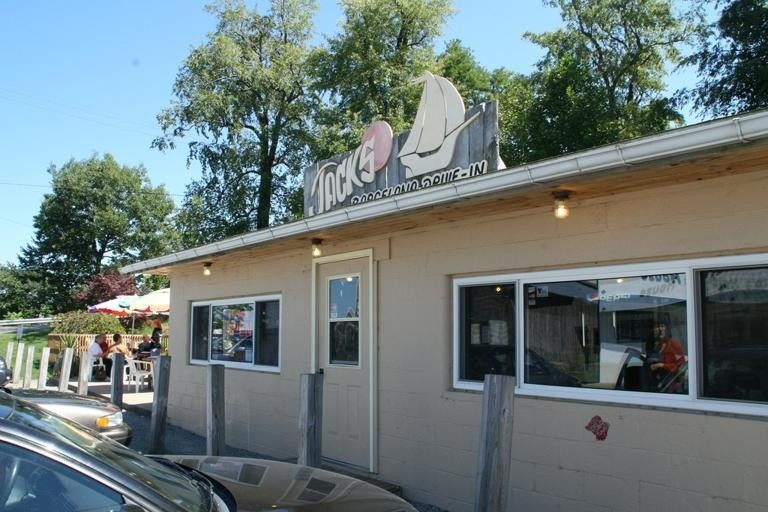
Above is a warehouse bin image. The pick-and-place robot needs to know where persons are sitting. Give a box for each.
[89,319,163,381]
[648,320,685,393]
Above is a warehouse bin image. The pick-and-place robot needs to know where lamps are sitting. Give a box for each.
[202,262,213,276]
[551,187,573,220]
[310,238,324,257]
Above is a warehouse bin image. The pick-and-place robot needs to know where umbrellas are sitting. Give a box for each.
[87,287,170,354]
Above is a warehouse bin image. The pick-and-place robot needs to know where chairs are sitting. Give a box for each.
[89,346,165,392]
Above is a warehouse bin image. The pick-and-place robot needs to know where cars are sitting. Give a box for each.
[612,346,689,395]
[465,343,580,388]
